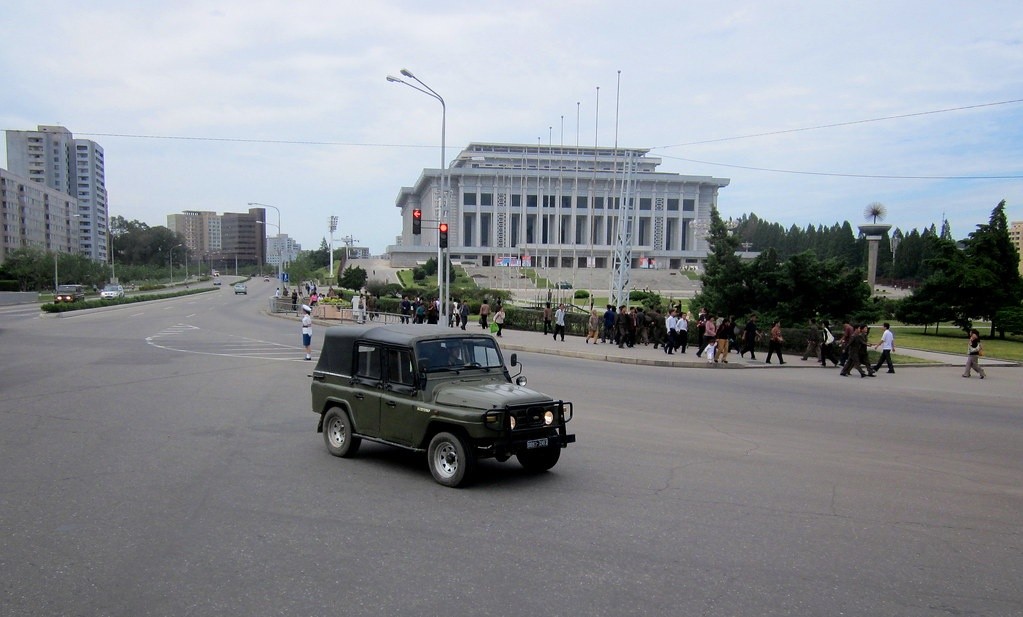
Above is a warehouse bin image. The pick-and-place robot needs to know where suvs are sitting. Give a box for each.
[54,285,84,303]
[101,284,125,300]
[310,321,576,486]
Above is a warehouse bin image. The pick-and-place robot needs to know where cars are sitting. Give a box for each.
[214,280,221,285]
[556,282,572,289]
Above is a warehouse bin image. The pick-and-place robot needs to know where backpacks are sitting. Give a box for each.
[979,343,984,355]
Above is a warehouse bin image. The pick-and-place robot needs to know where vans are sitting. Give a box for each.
[264,276,270,282]
[235,283,247,294]
[214,271,219,276]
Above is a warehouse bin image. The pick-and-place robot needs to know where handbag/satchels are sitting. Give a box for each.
[823,326,834,344]
[490,322,500,333]
[479,317,483,324]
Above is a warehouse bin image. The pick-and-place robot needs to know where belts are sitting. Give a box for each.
[303,325,308,327]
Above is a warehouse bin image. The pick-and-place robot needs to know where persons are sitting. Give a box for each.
[552,303,565,341]
[547,288,553,302]
[276,279,506,337]
[591,294,594,310]
[544,301,554,335]
[962,329,986,379]
[301,304,312,361]
[585,299,876,378]
[872,322,896,374]
[441,342,470,367]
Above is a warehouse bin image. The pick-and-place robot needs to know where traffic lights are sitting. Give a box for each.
[413,210,422,236]
[439,224,447,249]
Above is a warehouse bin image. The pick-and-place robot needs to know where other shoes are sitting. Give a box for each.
[586,338,588,343]
[304,356,312,360]
[840,366,895,377]
[664,347,674,353]
[980,375,984,378]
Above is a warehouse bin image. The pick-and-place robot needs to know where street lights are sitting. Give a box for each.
[186,247,197,281]
[170,244,182,282]
[199,251,209,281]
[386,69,445,322]
[54,215,80,291]
[112,234,120,278]
[209,252,238,276]
[255,256,262,276]
[248,201,284,295]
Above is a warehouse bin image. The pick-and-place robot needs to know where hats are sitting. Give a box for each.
[302,304,312,312]
